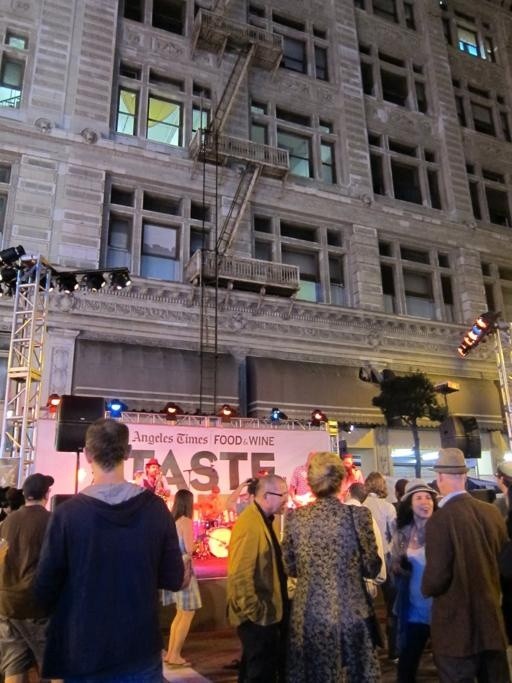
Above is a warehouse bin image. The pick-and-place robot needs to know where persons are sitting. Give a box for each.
[25,416,187,681]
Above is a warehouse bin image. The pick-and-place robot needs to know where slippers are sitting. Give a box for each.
[163,658,195,669]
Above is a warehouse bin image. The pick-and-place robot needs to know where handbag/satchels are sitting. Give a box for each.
[365,612,386,653]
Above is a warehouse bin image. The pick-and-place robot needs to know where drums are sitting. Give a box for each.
[206,525,231,559]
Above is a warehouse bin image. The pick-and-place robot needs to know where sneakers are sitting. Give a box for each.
[222,659,241,673]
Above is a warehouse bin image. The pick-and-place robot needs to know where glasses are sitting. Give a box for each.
[493,472,500,476]
[265,491,289,499]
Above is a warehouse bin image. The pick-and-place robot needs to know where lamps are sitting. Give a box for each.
[0,244,132,293]
[459,308,502,355]
[48,392,357,434]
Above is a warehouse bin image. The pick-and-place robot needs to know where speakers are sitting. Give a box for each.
[441,439,481,458]
[438,415,480,439]
[51,494,77,512]
[56,395,106,424]
[55,423,93,452]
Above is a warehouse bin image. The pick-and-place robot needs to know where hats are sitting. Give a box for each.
[145,458,162,467]
[399,478,439,503]
[22,472,55,501]
[426,447,471,474]
[0,486,24,507]
[496,461,512,483]
